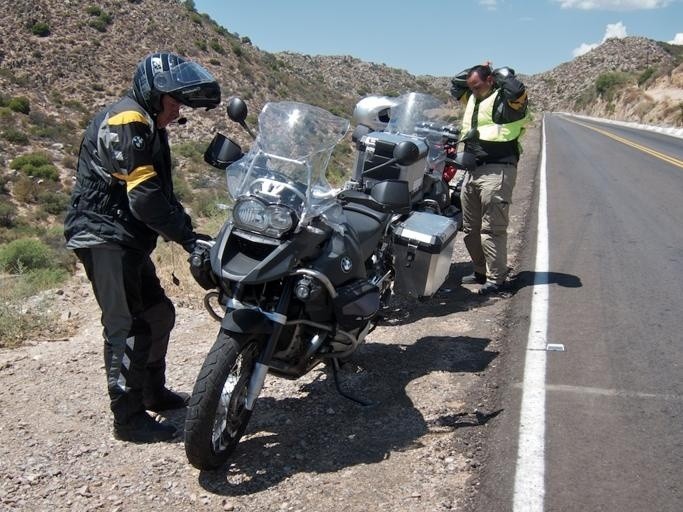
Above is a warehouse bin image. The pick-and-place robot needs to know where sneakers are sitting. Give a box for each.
[143,388,190,411]
[462,272,486,285]
[114,414,176,443]
[479,281,505,295]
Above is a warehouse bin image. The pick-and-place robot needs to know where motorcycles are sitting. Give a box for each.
[184,93,459,470]
[350,91,480,220]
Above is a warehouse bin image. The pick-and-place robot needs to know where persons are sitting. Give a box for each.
[63,52,220,440]
[448,62,527,298]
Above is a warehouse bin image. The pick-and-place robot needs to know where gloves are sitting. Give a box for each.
[183,231,211,253]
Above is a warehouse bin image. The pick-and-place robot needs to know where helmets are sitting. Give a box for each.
[129,53,221,123]
[352,96,400,133]
